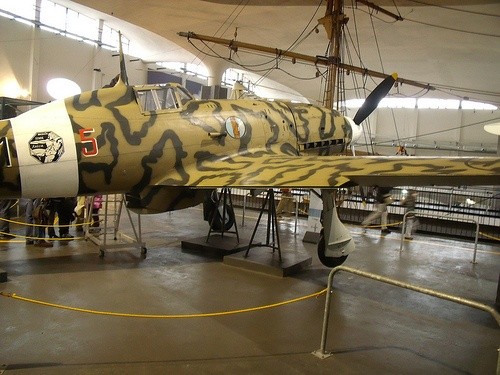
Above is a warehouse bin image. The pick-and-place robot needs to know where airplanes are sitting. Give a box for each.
[1,74,499,234]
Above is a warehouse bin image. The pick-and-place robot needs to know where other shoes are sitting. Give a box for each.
[1,234,15,240]
[77,227,83,232]
[91,223,99,227]
[34,239,53,247]
[60,234,74,241]
[26,240,34,244]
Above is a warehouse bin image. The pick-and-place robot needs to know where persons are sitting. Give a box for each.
[276,188,294,220]
[0,194,103,247]
[399,190,418,239]
[361,186,393,234]
